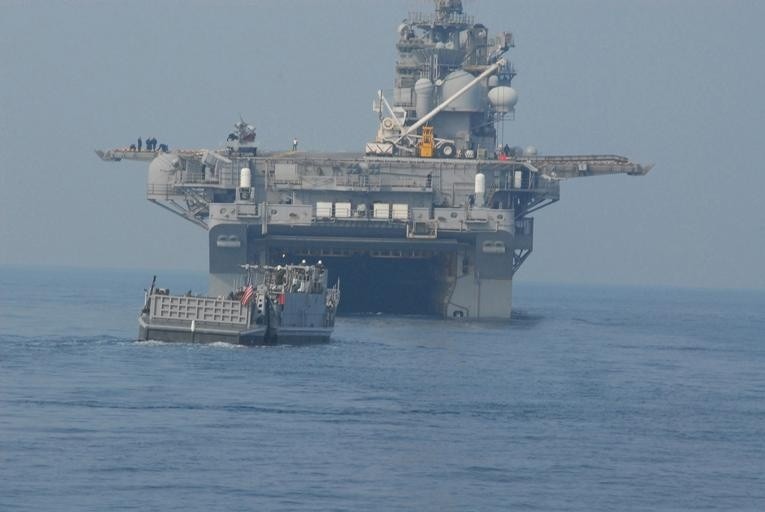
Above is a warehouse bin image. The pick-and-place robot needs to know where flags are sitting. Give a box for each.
[241,285,253,305]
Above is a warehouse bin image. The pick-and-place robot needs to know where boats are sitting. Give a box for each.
[138,254,342,347]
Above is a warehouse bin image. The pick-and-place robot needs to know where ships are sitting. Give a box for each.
[92,1,655,322]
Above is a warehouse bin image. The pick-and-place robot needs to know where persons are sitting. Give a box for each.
[129,136,168,151]
[292,138,299,151]
[503,144,510,156]
